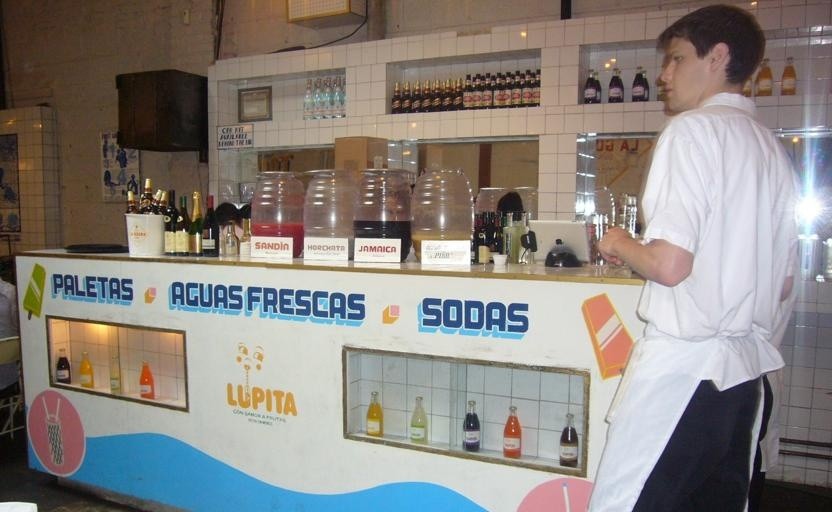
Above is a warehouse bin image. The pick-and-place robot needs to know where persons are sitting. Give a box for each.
[588,4,801,512]
[0,276,21,400]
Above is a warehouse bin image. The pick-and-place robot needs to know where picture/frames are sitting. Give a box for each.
[99,130,141,203]
[238,85,273,123]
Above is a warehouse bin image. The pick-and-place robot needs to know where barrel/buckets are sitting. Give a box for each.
[251,174,304,259]
[303,169,355,262]
[409,169,474,262]
[355,168,414,259]
[125,210,167,260]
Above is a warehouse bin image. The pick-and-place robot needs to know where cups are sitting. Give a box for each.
[43,416,67,466]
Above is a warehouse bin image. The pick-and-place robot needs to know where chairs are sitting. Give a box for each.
[0,335,24,438]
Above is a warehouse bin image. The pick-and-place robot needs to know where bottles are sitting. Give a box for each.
[743,56,797,97]
[125,176,168,216]
[55,346,159,403]
[583,66,666,102]
[166,186,250,262]
[392,69,541,114]
[475,206,531,268]
[589,180,638,264]
[365,385,580,469]
[302,75,347,118]
